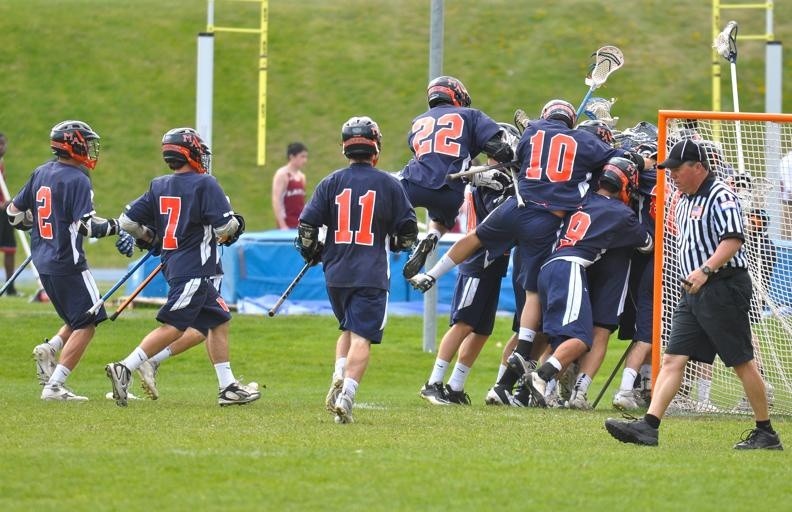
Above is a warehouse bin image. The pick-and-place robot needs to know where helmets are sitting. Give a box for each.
[425,76,472,108]
[600,157,639,192]
[47,120,99,167]
[340,117,381,158]
[160,128,211,174]
[576,119,615,148]
[539,100,575,130]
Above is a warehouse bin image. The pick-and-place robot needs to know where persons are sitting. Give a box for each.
[106,127,261,409]
[5,120,125,403]
[140,151,258,400]
[293,76,792,451]
[0,134,25,296]
[272,143,308,231]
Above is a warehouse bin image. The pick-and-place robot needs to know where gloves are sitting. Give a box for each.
[117,230,136,258]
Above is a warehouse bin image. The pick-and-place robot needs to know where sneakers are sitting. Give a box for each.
[604,418,660,445]
[613,378,717,411]
[403,232,439,292]
[219,385,258,406]
[421,381,471,406]
[333,396,354,424]
[41,386,89,403]
[736,430,783,451]
[734,382,771,412]
[139,360,162,403]
[34,344,57,384]
[106,362,131,408]
[326,376,344,410]
[485,366,590,411]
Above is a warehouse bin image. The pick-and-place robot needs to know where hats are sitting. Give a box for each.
[658,141,707,171]
